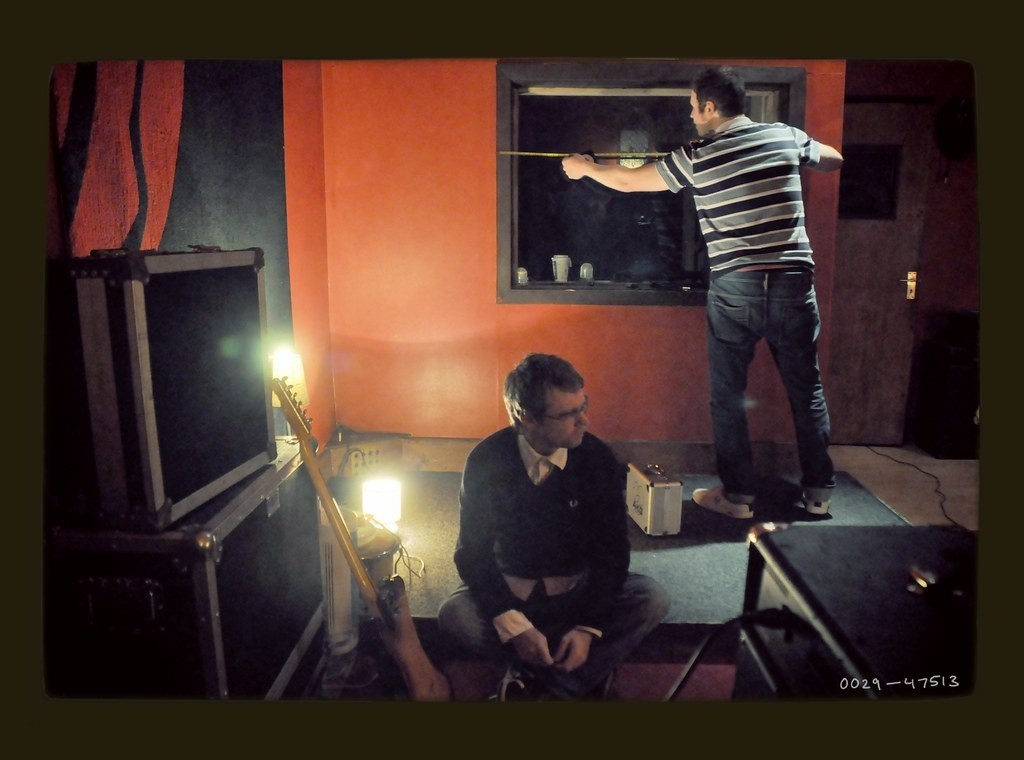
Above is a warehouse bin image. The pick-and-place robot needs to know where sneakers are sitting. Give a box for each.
[692,487,754,519]
[596,663,620,701]
[495,650,526,701]
[802,492,831,514]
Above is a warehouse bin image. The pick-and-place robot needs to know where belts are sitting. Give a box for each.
[735,261,806,272]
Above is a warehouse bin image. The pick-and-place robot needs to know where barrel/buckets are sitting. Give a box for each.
[316,508,359,656]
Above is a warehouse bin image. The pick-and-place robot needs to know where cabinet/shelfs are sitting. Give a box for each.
[729,524,975,699]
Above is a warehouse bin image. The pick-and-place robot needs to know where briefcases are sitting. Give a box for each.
[624,461,683,536]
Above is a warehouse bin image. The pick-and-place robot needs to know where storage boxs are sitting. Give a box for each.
[626,462,684,536]
[62,249,278,523]
[91,437,324,707]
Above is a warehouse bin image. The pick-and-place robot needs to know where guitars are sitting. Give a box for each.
[270,373,452,702]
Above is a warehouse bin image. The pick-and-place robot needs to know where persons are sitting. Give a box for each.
[437,352,670,702]
[563,65,844,519]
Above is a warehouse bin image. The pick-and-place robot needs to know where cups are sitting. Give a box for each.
[551,254,571,283]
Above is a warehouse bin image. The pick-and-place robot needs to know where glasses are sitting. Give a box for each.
[539,395,589,421]
